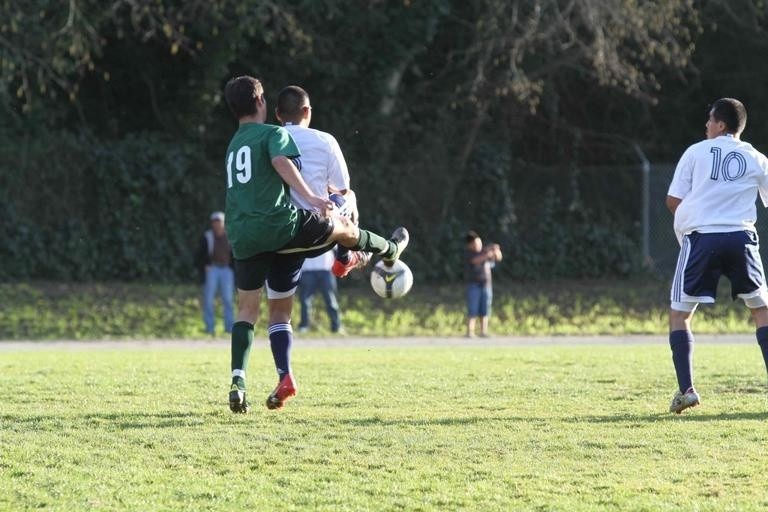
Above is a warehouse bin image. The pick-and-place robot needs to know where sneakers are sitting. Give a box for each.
[267,373,296,409]
[383,227,410,267]
[332,249,374,278]
[229,384,246,415]
[300,327,345,336]
[670,387,700,411]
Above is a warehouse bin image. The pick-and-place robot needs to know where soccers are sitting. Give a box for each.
[371,259,414,298]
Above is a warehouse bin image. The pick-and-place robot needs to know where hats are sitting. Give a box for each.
[211,212,226,221]
[466,232,479,241]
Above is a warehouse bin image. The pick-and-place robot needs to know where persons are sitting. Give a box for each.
[265,84,362,408]
[224,76,411,410]
[665,97,768,413]
[460,230,502,337]
[295,244,347,334]
[193,211,235,336]
